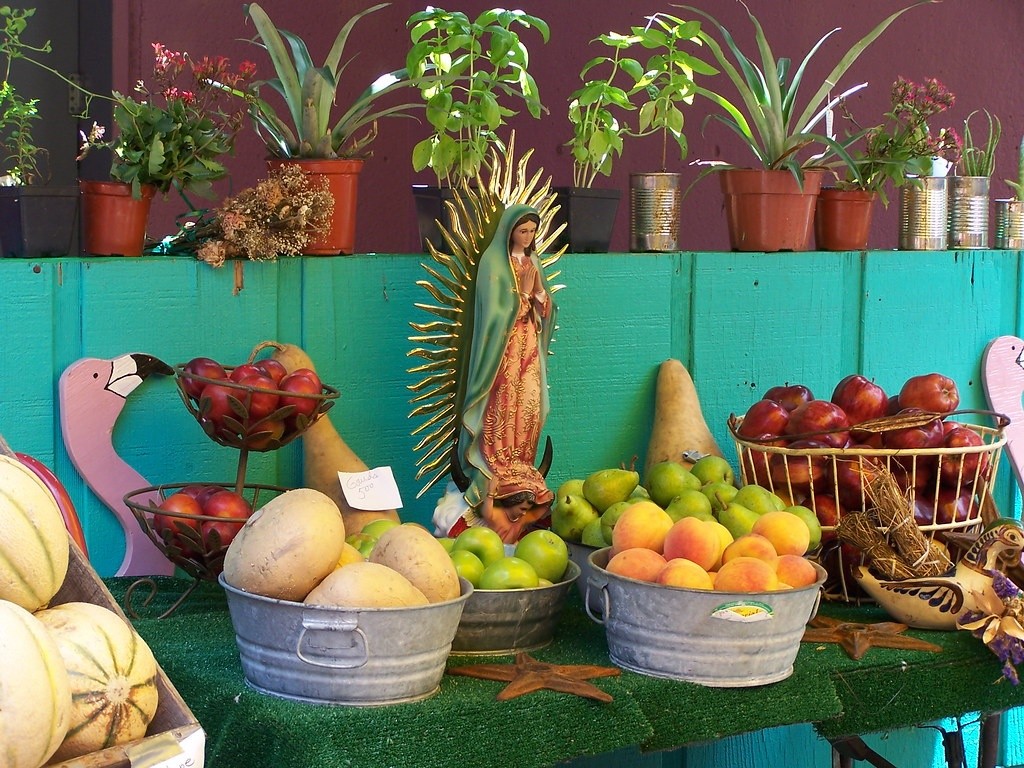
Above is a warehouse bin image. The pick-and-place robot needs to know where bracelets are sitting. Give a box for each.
[486,492,498,498]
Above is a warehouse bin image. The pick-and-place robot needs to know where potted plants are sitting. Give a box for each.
[883,105,949,252]
[946,107,1001,250]
[643,0,924,252]
[995,136,1024,250]
[406,6,550,255]
[548,15,721,254]
[200,2,550,254]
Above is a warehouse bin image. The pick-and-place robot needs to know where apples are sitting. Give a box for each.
[347,519,568,590]
[737,373,993,590]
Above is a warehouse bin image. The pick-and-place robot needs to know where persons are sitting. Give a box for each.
[480,476,553,544]
[458,204,554,507]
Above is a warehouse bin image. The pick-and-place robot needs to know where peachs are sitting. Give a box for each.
[605,501,817,591]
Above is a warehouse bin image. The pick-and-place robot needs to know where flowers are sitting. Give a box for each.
[0,5,121,186]
[73,42,256,202]
[835,74,962,211]
[144,163,336,271]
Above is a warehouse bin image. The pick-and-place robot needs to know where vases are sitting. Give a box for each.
[79,183,157,256]
[814,186,880,252]
[0,186,80,258]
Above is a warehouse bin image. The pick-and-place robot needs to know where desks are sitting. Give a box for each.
[100,575,1023,768]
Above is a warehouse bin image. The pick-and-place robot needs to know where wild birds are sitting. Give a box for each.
[981,336,1024,501]
[849,517,1024,632]
[59,353,176,577]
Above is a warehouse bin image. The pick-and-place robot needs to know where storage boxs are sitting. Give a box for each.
[0,435,205,768]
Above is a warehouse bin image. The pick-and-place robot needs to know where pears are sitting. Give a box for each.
[551,456,822,549]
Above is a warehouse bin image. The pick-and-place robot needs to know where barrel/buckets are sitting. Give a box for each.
[587,546,828,689]
[452,543,581,657]
[564,540,608,612]
[218,572,476,708]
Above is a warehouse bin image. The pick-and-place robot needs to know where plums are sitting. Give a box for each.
[184,358,322,447]
[154,483,253,554]
